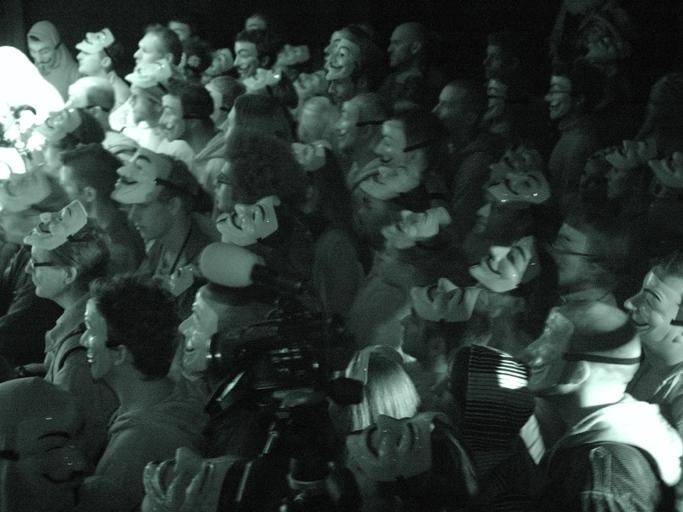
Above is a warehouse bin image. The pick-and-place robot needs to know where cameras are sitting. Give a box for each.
[199,242,368,493]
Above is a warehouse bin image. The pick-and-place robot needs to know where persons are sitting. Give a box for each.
[1,0,683,510]
